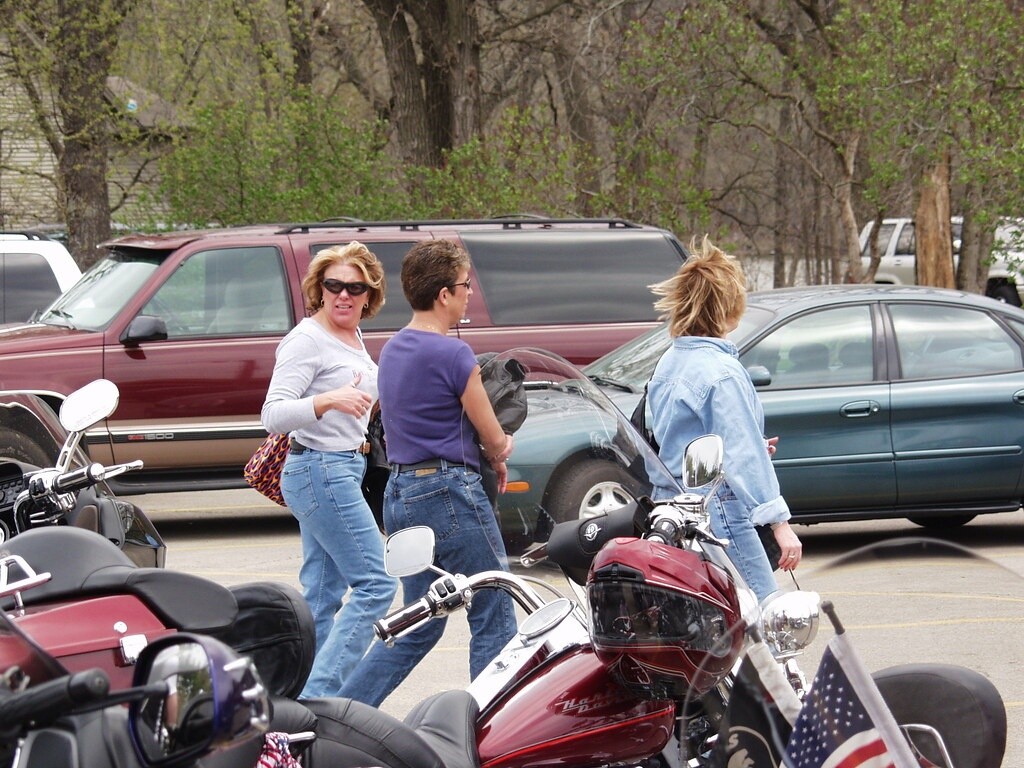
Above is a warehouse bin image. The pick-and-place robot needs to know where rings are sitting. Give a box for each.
[789,555,795,558]
[349,381,356,388]
[504,458,509,462]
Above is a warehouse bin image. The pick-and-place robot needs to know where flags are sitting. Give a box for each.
[778,631,920,768]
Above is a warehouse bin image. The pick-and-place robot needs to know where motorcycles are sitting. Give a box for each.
[0,517,821,768]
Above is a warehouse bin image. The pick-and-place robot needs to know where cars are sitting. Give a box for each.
[477,285,1024,556]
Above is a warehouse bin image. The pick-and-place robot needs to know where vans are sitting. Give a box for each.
[842,216,1024,308]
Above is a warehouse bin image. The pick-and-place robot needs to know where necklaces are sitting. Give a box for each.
[410,321,440,331]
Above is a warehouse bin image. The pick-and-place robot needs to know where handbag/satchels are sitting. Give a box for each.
[244,429,291,507]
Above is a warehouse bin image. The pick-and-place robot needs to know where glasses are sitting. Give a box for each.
[449,278,471,289]
[320,277,369,295]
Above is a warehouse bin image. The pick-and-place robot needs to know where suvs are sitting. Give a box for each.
[0,231,84,324]
[0,214,691,495]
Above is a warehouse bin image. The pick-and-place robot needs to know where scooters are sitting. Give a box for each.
[373,345,730,714]
[0,632,274,768]
[0,378,167,570]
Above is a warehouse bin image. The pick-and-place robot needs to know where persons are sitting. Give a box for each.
[643,229,803,606]
[333,237,517,709]
[258,238,399,703]
